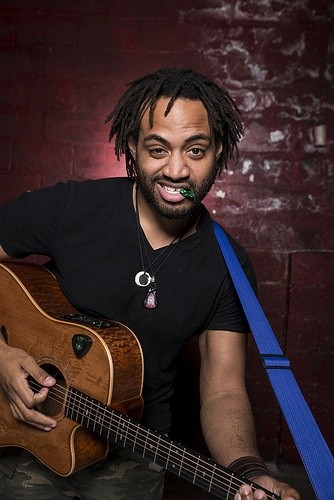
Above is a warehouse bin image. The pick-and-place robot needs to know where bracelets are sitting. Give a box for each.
[227,457,269,481]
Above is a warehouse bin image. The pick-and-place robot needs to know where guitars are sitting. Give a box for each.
[0,263,283,500]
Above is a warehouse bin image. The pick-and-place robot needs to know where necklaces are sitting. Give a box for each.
[134,183,195,309]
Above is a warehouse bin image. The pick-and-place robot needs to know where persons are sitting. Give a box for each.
[1,67,299,500]
